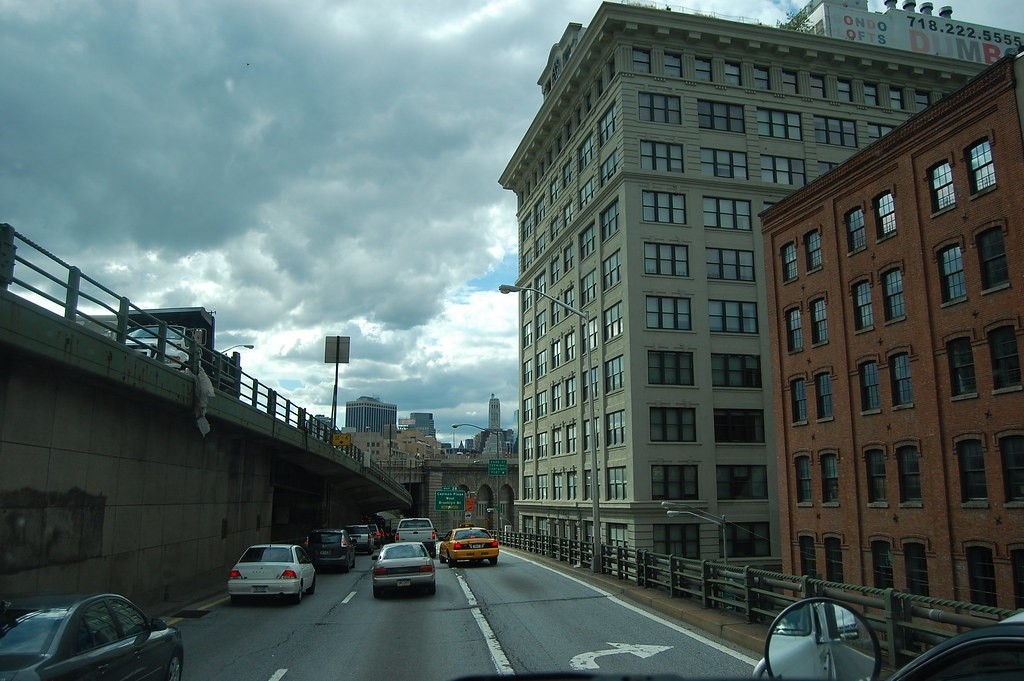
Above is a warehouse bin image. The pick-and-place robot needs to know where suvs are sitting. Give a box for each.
[302,529,357,574]
[344,524,375,555]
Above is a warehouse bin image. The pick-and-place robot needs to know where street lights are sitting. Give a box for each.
[660,500,730,587]
[497,282,601,574]
[452,423,500,543]
[365,426,372,458]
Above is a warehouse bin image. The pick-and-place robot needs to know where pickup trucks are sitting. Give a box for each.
[391,517,438,559]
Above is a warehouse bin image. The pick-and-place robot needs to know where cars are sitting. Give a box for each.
[438,522,500,568]
[371,540,437,598]
[113,323,202,375]
[226,543,316,604]
[0,588,185,681]
[368,524,386,549]
